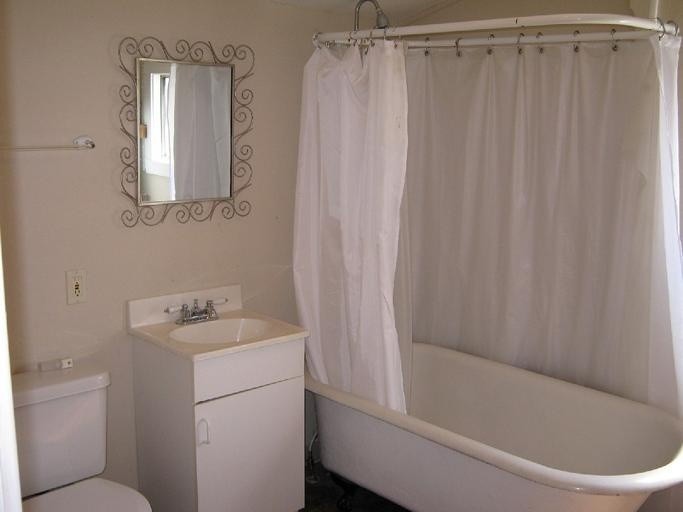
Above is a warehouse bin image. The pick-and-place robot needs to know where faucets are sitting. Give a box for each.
[189,297,205,326]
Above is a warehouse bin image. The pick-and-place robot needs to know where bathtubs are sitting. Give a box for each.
[305,340,683,511]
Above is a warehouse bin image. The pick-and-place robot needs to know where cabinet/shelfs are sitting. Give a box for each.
[135,375,307,512]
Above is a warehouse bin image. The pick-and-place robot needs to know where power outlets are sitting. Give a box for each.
[64,268,87,307]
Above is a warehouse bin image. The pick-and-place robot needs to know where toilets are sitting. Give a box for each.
[11,364,156,512]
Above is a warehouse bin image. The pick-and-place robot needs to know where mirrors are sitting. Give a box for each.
[114,34,257,232]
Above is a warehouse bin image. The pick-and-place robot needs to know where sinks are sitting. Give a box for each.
[168,317,272,346]
